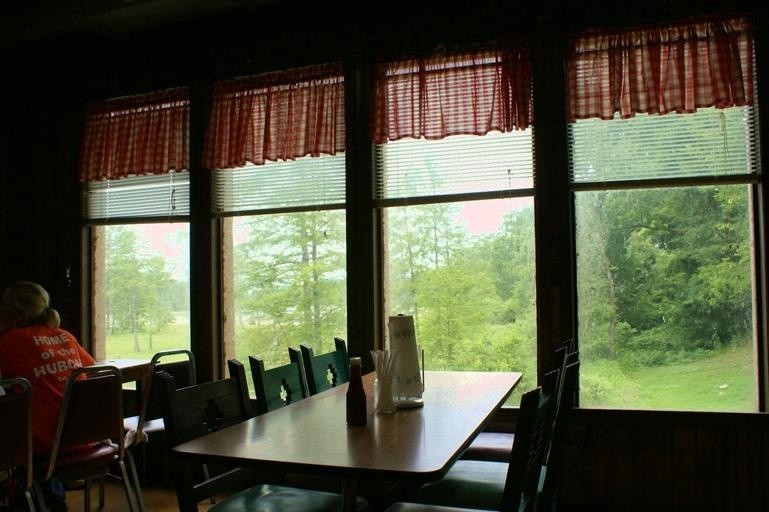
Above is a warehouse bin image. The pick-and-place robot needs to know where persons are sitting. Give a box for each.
[1,281,99,511]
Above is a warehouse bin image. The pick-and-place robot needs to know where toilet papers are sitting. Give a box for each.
[388,315,423,400]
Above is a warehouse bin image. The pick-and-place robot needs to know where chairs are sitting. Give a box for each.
[2,378,37,510]
[162,361,372,512]
[35,366,137,507]
[99,350,196,511]
[388,342,577,511]
[248,340,347,416]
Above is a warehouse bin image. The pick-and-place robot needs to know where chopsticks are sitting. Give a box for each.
[369,348,398,379]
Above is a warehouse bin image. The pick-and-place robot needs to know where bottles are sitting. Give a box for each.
[374,380,396,416]
[345,357,368,427]
